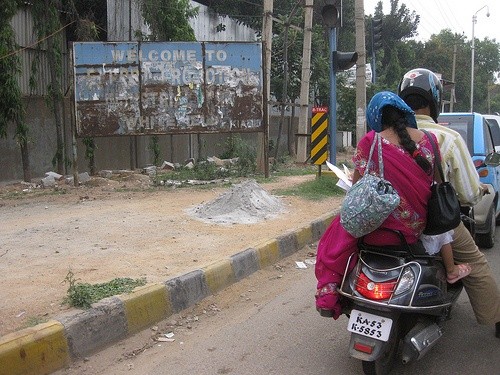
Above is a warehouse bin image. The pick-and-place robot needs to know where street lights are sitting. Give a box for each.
[470,4,490,113]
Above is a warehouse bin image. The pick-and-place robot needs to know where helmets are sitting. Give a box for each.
[398,68,442,118]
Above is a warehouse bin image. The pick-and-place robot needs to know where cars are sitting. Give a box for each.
[436,111,500,249]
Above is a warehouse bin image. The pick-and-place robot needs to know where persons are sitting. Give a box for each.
[314,69,500,338]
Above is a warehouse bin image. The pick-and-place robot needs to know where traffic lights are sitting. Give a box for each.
[370,18,383,51]
[332,51,359,72]
[319,0,343,28]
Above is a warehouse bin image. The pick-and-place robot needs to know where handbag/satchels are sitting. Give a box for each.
[422,127,461,235]
[340,132,400,238]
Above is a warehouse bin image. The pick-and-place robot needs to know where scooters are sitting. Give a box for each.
[334,150,500,375]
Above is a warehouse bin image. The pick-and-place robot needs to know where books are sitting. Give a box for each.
[325,159,353,191]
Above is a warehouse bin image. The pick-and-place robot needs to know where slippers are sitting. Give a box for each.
[447,263,472,284]
